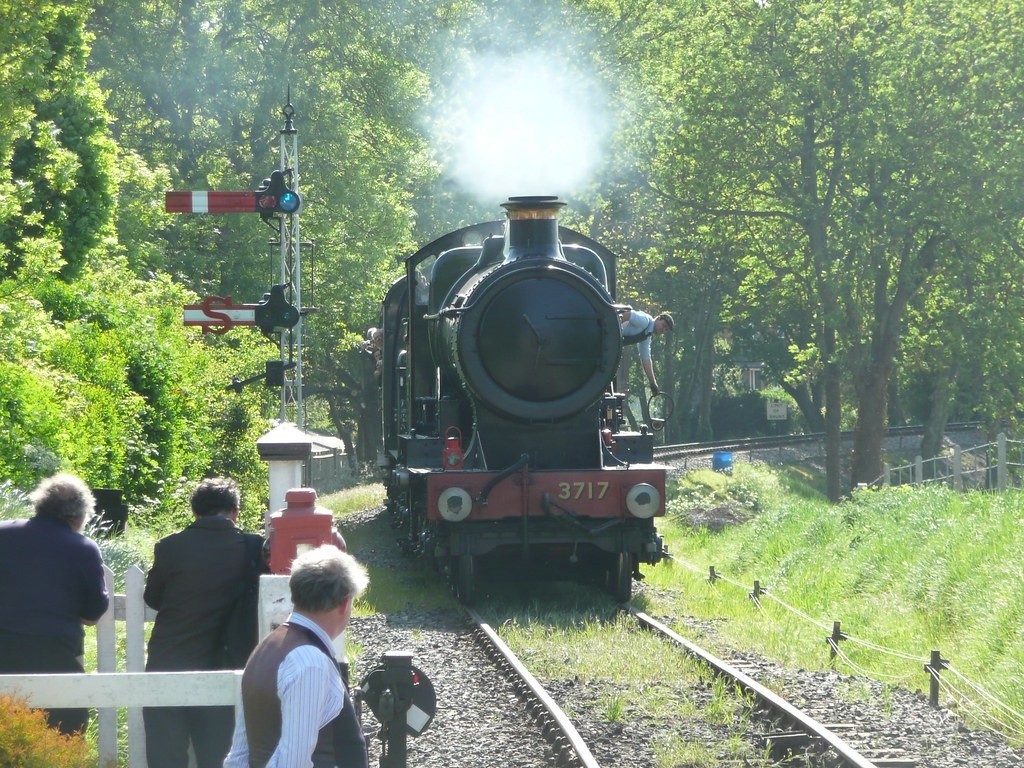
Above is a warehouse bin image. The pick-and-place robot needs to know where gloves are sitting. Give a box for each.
[651,383,659,397]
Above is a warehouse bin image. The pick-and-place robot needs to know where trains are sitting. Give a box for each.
[375,192,673,609]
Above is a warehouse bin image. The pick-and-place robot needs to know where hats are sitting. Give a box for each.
[660,314,674,331]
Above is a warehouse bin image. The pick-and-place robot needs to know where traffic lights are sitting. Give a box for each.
[252,283,301,334]
[264,360,284,388]
[254,169,300,214]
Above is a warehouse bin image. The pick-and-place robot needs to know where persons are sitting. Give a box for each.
[223,545,370,768]
[143,476,270,768]
[619,309,674,397]
[0,472,109,742]
[397,334,407,367]
[358,327,383,392]
